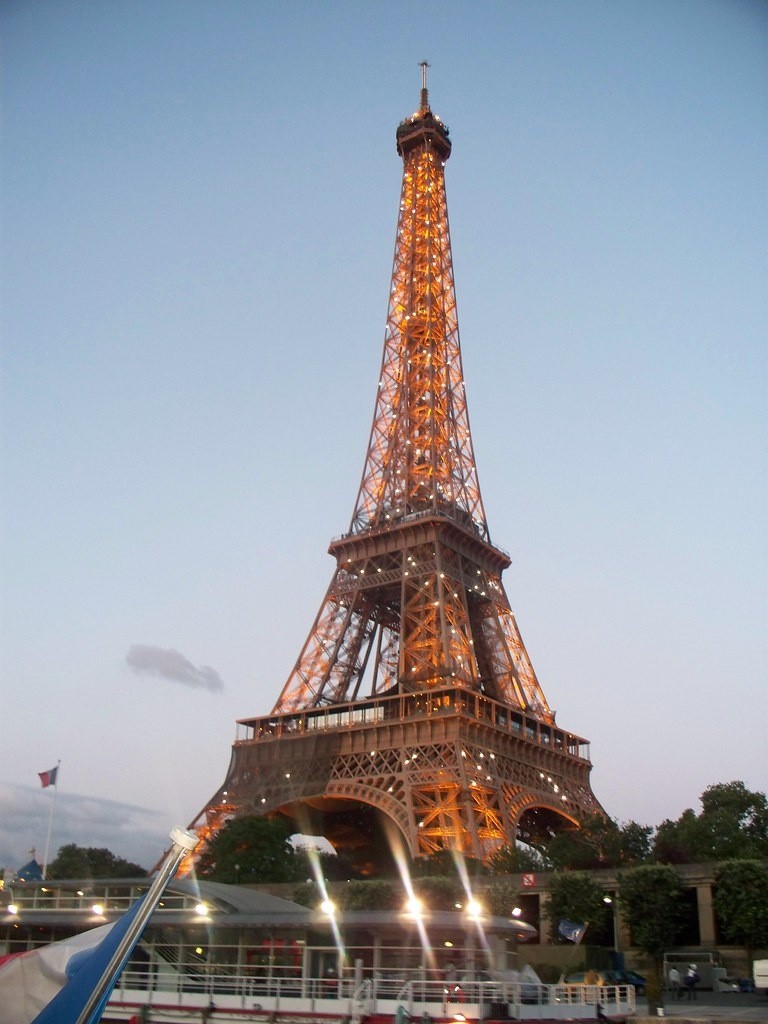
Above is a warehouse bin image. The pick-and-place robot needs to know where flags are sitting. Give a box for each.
[556,919,584,944]
[38,766,59,789]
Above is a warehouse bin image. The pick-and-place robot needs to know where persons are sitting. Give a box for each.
[442,960,458,988]
[668,966,682,1002]
[684,965,699,1001]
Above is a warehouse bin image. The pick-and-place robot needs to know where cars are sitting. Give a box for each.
[565,967,645,996]
